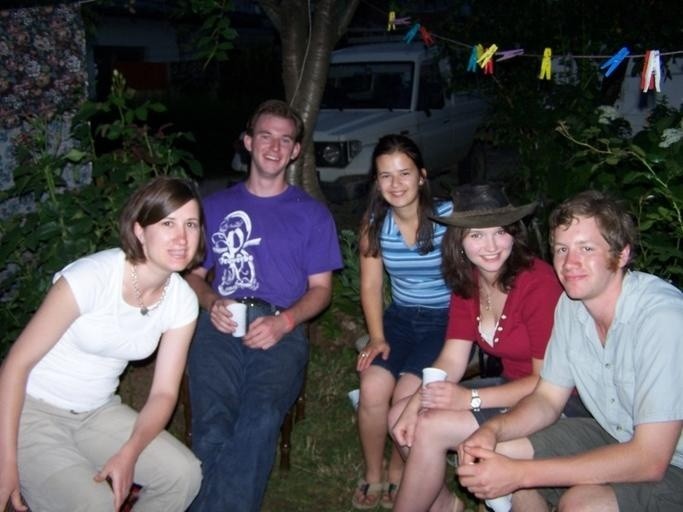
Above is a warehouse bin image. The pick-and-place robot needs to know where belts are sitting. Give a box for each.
[231,296,280,311]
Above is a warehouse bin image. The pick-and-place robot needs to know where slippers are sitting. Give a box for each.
[381,477,402,509]
[351,470,383,510]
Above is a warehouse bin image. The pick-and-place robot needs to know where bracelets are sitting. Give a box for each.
[278,307,297,332]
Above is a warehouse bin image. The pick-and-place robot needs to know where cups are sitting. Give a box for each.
[421,367,447,387]
[226,304,248,337]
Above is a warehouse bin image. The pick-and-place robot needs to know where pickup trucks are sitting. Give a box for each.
[227,37,498,200]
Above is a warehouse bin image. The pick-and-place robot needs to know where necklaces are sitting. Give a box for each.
[129,263,171,318]
[480,281,500,314]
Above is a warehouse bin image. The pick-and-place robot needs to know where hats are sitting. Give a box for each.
[425,181,541,231]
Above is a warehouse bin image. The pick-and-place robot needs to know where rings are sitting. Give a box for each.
[361,352,367,358]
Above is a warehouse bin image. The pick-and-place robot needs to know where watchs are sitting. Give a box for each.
[468,388,483,415]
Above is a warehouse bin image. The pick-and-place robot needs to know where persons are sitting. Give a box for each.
[458,189,683,511]
[1,176,208,512]
[179,102,346,512]
[385,185,564,511]
[349,135,458,510]
